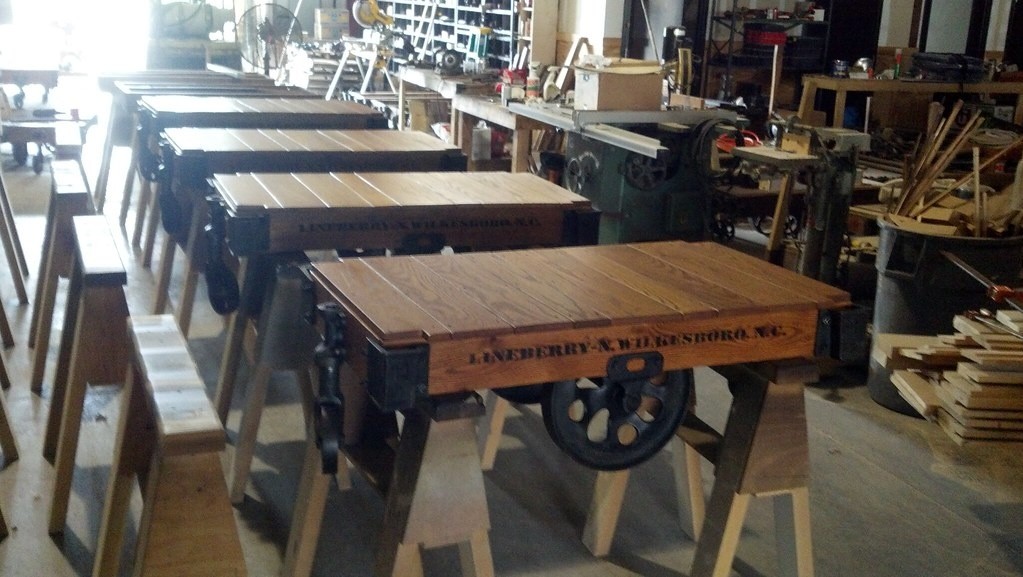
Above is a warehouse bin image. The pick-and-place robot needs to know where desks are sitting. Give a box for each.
[144,123,469,338]
[209,163,603,506]
[280,238,852,577]
[121,95,400,243]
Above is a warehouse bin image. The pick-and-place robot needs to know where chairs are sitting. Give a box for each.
[30,160,99,349]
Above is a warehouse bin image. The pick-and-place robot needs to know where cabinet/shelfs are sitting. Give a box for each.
[700,0,830,126]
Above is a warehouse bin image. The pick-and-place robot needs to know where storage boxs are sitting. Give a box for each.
[742,23,789,44]
[572,57,670,112]
[472,125,492,161]
[314,23,350,40]
[315,8,349,24]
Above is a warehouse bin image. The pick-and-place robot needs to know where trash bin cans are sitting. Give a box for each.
[910,51,997,123]
[865,214,1023,419]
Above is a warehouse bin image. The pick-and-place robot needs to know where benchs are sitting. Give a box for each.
[48,118,95,224]
[92,313,251,577]
[45,213,146,536]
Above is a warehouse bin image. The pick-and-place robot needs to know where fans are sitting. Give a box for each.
[235,3,303,78]
